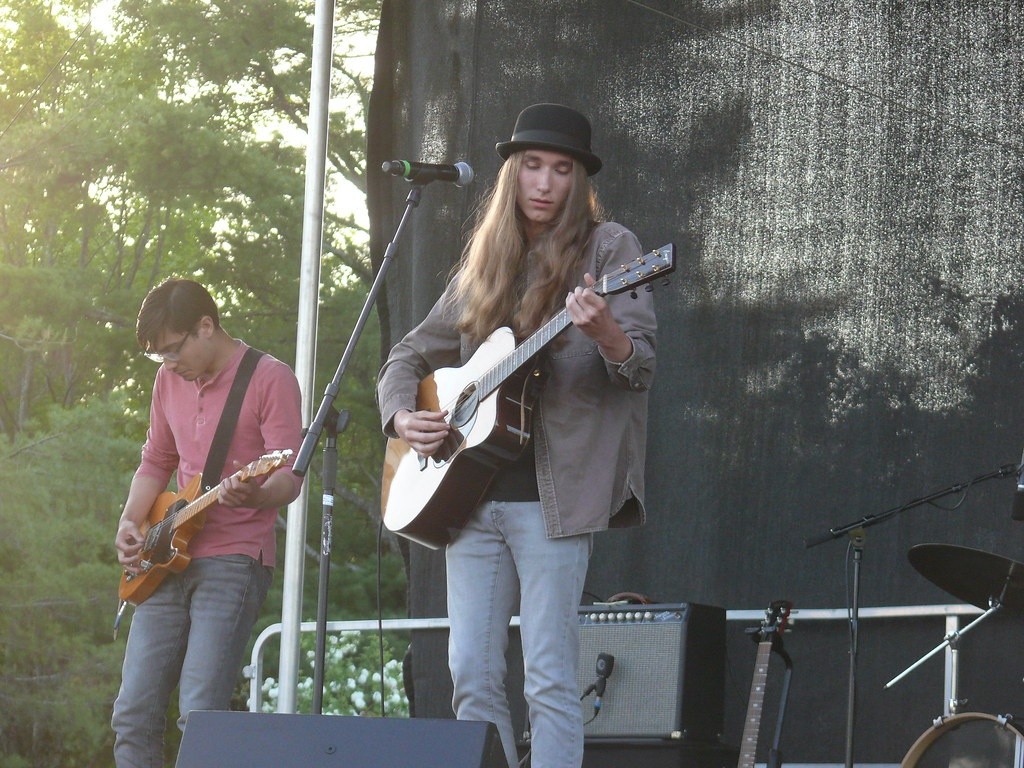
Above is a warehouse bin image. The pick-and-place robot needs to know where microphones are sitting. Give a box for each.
[1012,447,1024,522]
[593,652,615,718]
[381,160,473,188]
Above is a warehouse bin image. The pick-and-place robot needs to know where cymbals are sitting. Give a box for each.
[906,543,1024,617]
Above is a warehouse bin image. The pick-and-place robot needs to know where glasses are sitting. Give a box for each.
[144,327,198,362]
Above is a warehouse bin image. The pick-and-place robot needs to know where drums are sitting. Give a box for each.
[900,711,1024,768]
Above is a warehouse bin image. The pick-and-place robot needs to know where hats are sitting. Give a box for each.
[496,103,602,176]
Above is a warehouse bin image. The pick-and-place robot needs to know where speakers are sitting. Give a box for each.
[522,601,725,741]
[175,710,510,768]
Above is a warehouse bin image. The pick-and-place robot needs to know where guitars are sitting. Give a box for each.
[118,449,293,607]
[736,599,798,768]
[381,242,676,551]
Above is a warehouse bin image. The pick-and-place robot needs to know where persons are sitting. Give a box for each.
[112,279,304,768]
[376,105,658,768]
